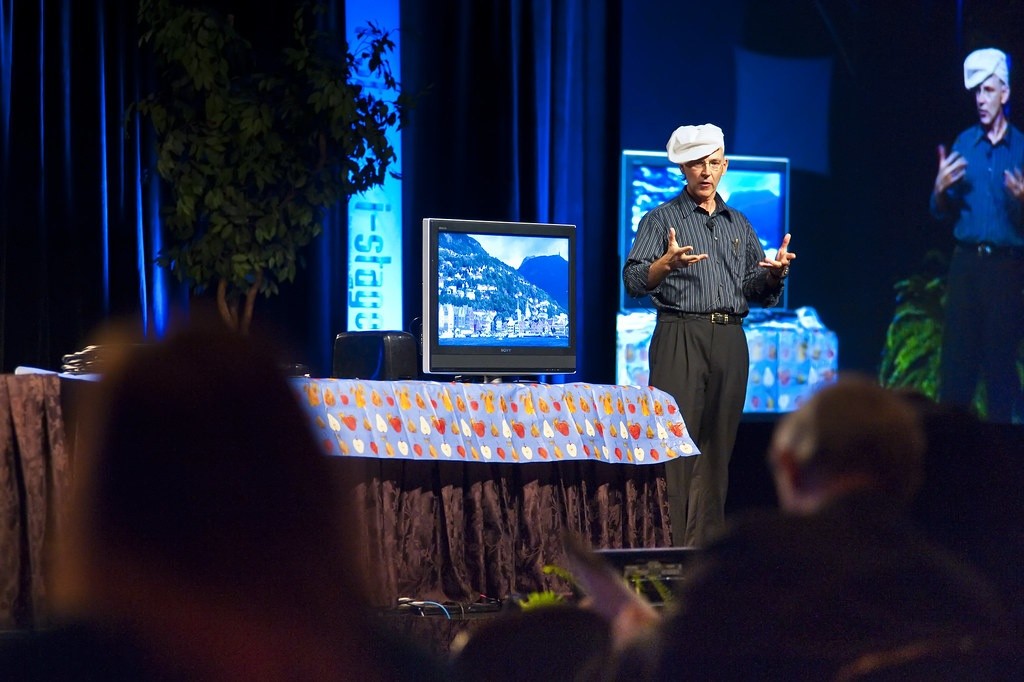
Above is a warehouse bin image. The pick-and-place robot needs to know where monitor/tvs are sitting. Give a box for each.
[423,217,578,375]
[618,151,791,315]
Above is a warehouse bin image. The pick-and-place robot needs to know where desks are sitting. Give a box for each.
[0,371,705,629]
[615,317,842,421]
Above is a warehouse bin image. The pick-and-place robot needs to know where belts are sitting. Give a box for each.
[955,240,1023,259]
[662,308,744,325]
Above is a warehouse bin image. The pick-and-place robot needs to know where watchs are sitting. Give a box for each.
[770,265,789,279]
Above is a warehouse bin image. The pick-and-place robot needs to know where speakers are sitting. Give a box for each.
[332,330,418,381]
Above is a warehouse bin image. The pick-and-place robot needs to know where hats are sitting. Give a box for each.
[665,123,725,165]
[964,48,1007,91]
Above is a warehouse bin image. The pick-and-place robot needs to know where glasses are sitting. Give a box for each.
[689,160,724,171]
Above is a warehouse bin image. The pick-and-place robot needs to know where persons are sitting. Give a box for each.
[43,315,1024,681]
[928,46,1024,423]
[617,123,797,551]
